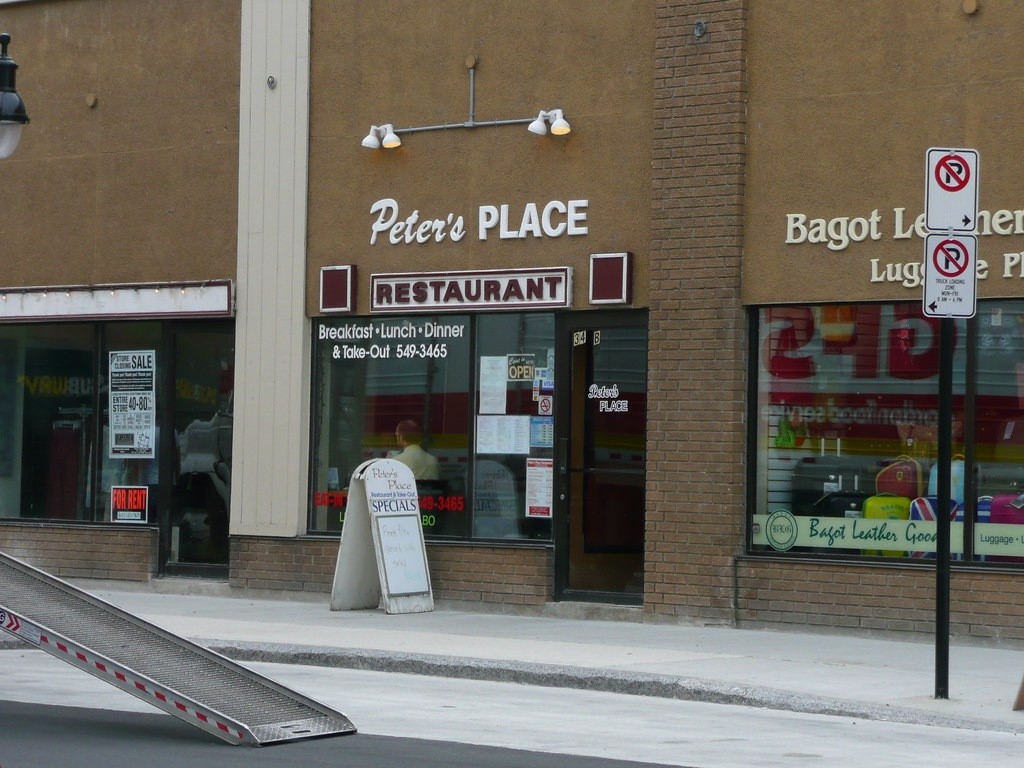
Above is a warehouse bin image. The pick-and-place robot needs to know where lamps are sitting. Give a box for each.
[527,108,572,136]
[360,123,402,150]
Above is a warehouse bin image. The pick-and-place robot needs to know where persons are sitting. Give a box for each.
[390,419,440,479]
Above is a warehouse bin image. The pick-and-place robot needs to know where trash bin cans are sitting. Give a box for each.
[790,455,867,518]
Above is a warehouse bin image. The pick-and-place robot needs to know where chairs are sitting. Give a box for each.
[415,479,451,535]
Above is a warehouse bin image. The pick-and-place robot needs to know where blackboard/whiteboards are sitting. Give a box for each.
[375,512,431,597]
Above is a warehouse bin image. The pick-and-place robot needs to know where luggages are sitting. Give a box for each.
[791,427,1024,563]
